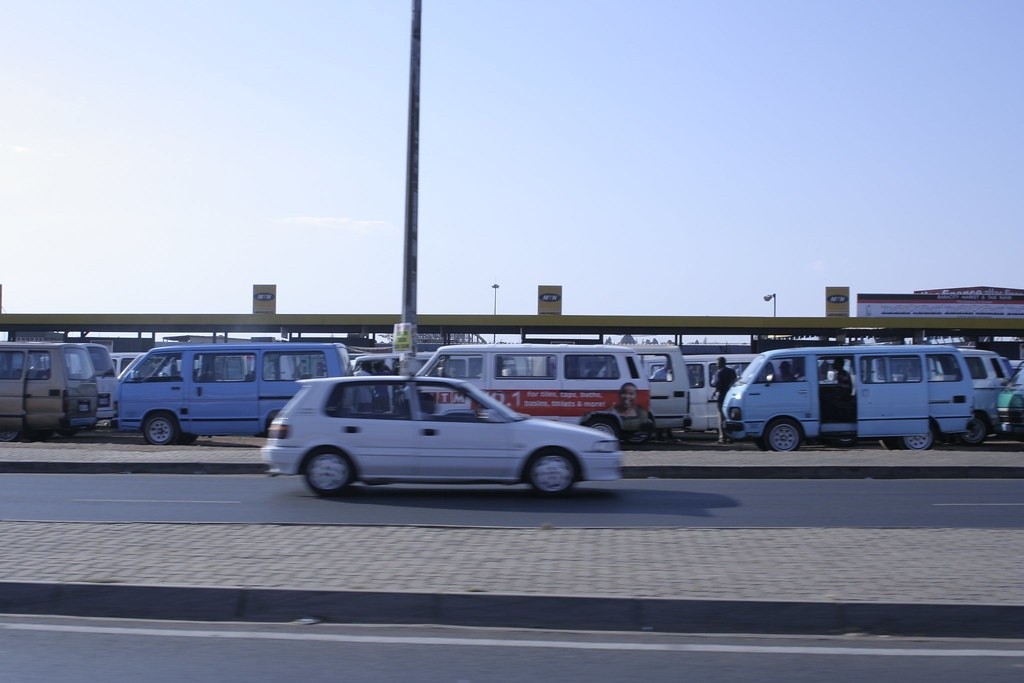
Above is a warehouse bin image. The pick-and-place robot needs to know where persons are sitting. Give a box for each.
[354,360,392,376]
[419,393,436,414]
[710,356,738,444]
[779,362,797,380]
[823,357,853,418]
[651,360,670,382]
[608,382,648,429]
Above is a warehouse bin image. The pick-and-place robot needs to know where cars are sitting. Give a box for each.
[261,373,624,499]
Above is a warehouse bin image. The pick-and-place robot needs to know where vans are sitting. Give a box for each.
[0,341,100,443]
[349,351,530,416]
[834,346,1024,439]
[25,343,201,425]
[395,342,654,441]
[115,342,355,446]
[719,343,975,452]
[597,353,763,434]
[535,344,694,435]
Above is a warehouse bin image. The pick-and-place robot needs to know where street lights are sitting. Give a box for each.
[491,283,500,341]
[764,292,776,338]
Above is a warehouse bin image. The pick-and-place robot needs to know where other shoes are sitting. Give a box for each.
[726,438,734,443]
[718,438,725,443]
